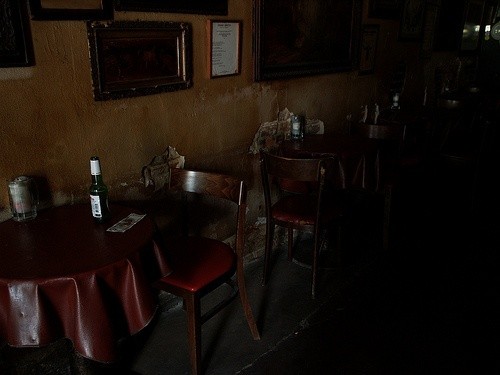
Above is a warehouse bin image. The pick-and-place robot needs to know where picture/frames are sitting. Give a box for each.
[367,0,405,21]
[204,18,242,79]
[416,3,440,60]
[29,0,114,21]
[0,0,35,68]
[86,20,194,101]
[114,0,229,16]
[456,0,486,57]
[357,24,380,76]
[252,0,360,82]
[398,0,425,42]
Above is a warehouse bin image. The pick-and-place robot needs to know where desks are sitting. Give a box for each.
[274,133,393,267]
[0,198,173,375]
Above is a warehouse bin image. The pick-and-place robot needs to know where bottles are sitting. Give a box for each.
[88,156,112,223]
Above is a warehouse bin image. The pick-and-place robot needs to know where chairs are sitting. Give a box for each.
[258,148,345,301]
[352,124,401,248]
[158,168,261,375]
